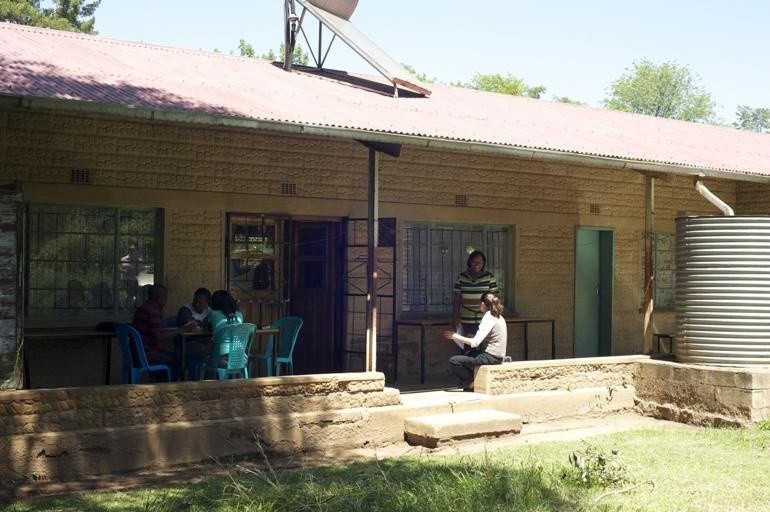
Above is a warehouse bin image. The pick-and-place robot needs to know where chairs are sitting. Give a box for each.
[114,315,304,384]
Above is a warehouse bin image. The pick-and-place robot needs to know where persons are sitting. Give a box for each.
[201,288,244,381]
[451,250,499,388]
[53,278,83,311]
[172,286,213,382]
[86,282,114,309]
[442,292,508,392]
[129,284,190,383]
[119,245,146,305]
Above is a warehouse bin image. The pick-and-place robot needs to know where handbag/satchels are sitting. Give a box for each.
[463,342,485,357]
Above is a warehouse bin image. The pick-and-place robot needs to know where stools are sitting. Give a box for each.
[653,333,676,354]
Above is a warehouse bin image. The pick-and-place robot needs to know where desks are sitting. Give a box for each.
[394,319,555,381]
